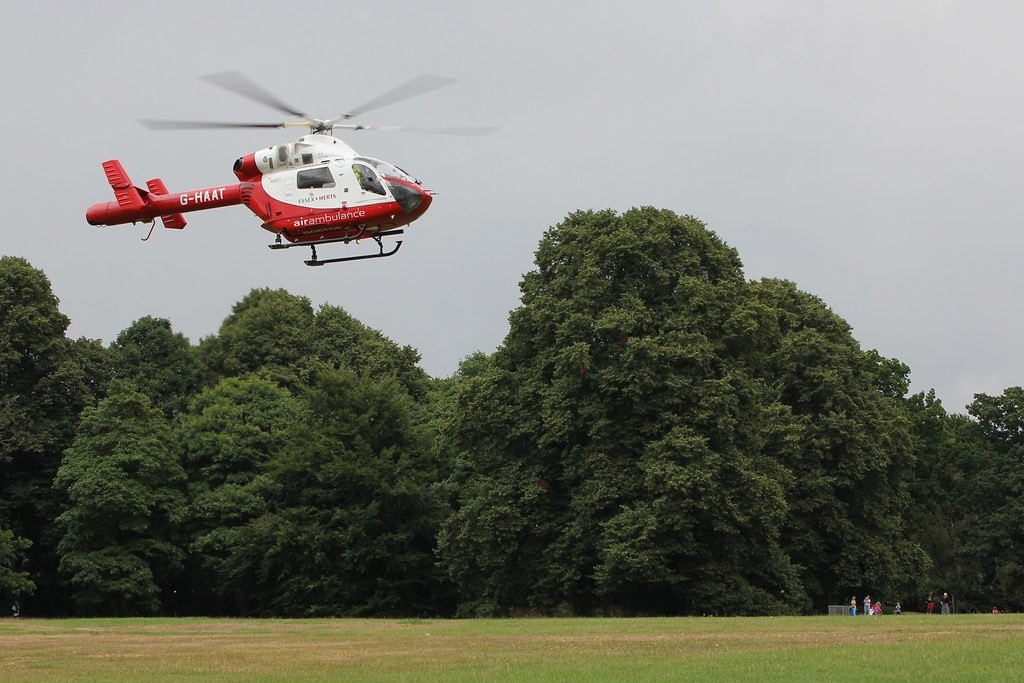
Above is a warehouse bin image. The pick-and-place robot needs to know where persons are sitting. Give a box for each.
[13,600,19,617]
[895,602,902,614]
[850,595,856,615]
[872,602,882,613]
[925,590,934,612]
[864,594,870,615]
[939,592,949,614]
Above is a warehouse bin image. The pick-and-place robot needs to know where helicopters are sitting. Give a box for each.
[85,72,445,266]
